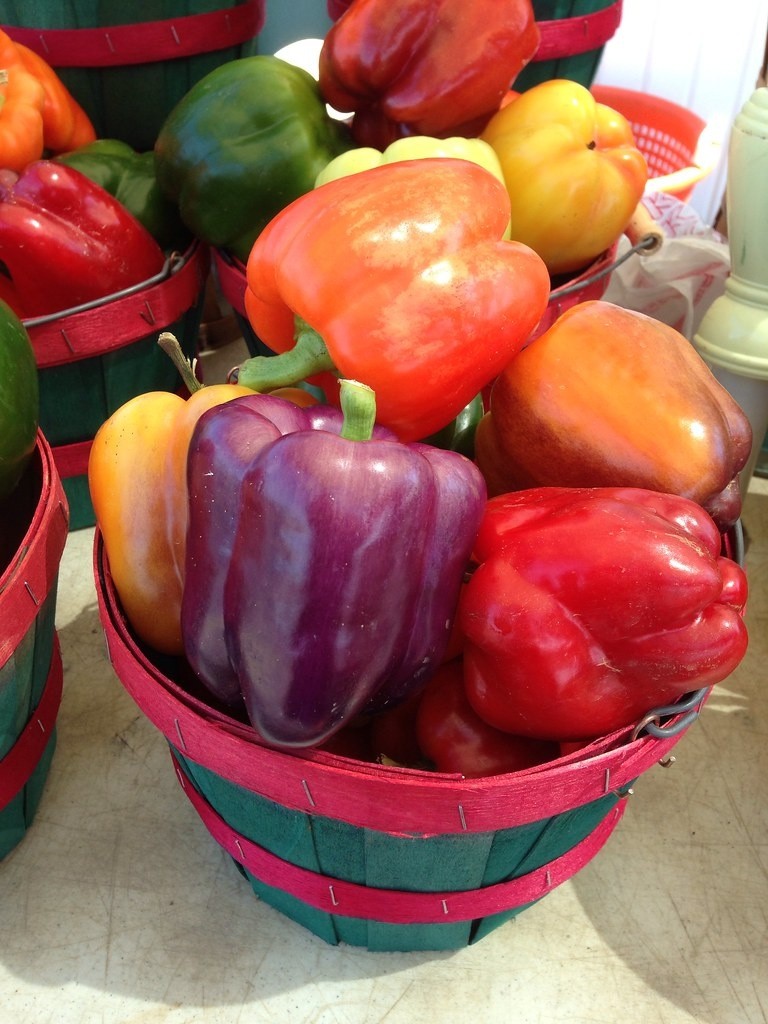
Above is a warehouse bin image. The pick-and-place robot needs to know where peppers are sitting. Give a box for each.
[0,0,751,778]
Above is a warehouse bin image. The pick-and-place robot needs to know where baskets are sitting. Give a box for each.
[509,81,710,345]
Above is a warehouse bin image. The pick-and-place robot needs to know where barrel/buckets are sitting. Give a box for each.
[326,2,624,95]
[1,427,71,864]
[215,201,665,413]
[22,240,208,533]
[0,2,267,154]
[94,521,746,954]
